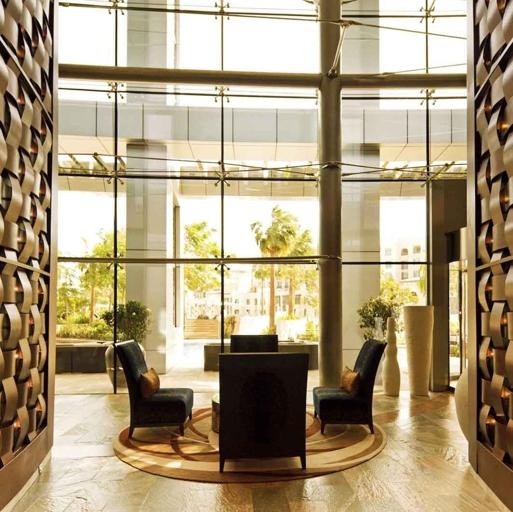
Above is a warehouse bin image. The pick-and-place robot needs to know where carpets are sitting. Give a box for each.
[112,407,388,484]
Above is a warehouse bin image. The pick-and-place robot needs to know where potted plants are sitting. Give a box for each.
[99,299,153,389]
[357,296,405,386]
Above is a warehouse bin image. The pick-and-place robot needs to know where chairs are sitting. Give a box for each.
[112,334,389,474]
